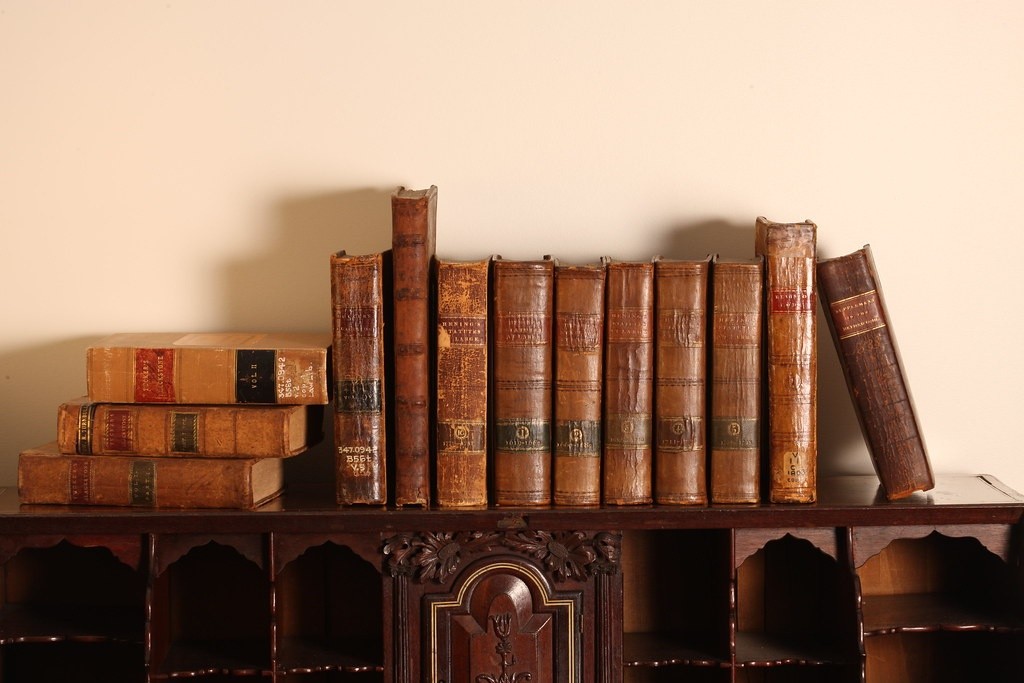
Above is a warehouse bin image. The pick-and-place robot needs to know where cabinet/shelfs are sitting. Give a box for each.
[0,460,1024,681]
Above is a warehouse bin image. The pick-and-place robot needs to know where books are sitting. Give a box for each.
[482,248,553,512]
[15,437,290,509]
[551,256,603,507]
[598,256,657,503]
[433,253,490,513]
[650,254,711,505]
[816,240,935,501]
[327,249,394,506]
[54,393,327,458]
[388,184,437,510]
[85,331,336,408]
[705,254,767,506]
[755,212,820,504]
